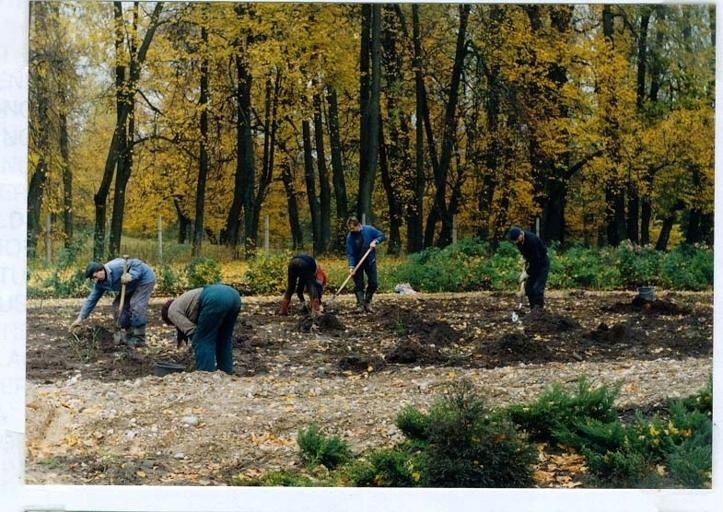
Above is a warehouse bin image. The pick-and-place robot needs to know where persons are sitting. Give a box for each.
[278,254,327,317]
[346,216,385,314]
[161,284,241,376]
[70,258,156,348]
[507,227,549,310]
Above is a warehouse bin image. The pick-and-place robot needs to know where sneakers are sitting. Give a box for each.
[127,335,147,346]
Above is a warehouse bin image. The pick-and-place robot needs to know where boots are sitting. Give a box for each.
[310,297,324,319]
[354,290,364,313]
[364,288,377,313]
[279,299,291,315]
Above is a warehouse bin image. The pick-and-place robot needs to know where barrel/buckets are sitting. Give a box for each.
[637,287,654,300]
[154,362,186,378]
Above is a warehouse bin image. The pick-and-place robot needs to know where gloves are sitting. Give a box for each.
[121,273,133,284]
[69,317,84,333]
[517,271,530,283]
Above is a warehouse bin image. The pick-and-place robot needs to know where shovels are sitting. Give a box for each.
[112,255,130,346]
[324,247,373,306]
[511,260,527,322]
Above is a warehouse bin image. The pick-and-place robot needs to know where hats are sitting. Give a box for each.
[85,262,104,278]
